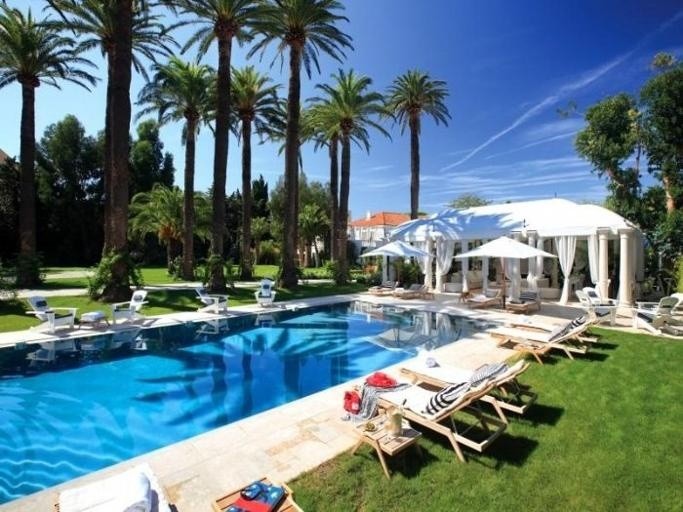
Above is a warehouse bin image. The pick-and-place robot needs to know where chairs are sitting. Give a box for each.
[24,313,280,364]
[338,372,508,464]
[21,277,284,337]
[49,461,176,512]
[358,267,437,310]
[396,348,537,417]
[442,264,683,368]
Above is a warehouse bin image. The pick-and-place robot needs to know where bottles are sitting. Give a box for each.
[135,472,152,511]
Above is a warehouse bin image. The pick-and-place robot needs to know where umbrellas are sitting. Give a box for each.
[452,236,557,308]
[357,239,436,282]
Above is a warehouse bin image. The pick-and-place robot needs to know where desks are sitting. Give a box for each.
[350,412,428,479]
[210,470,303,512]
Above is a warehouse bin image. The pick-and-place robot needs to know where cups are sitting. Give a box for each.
[385,410,403,441]
